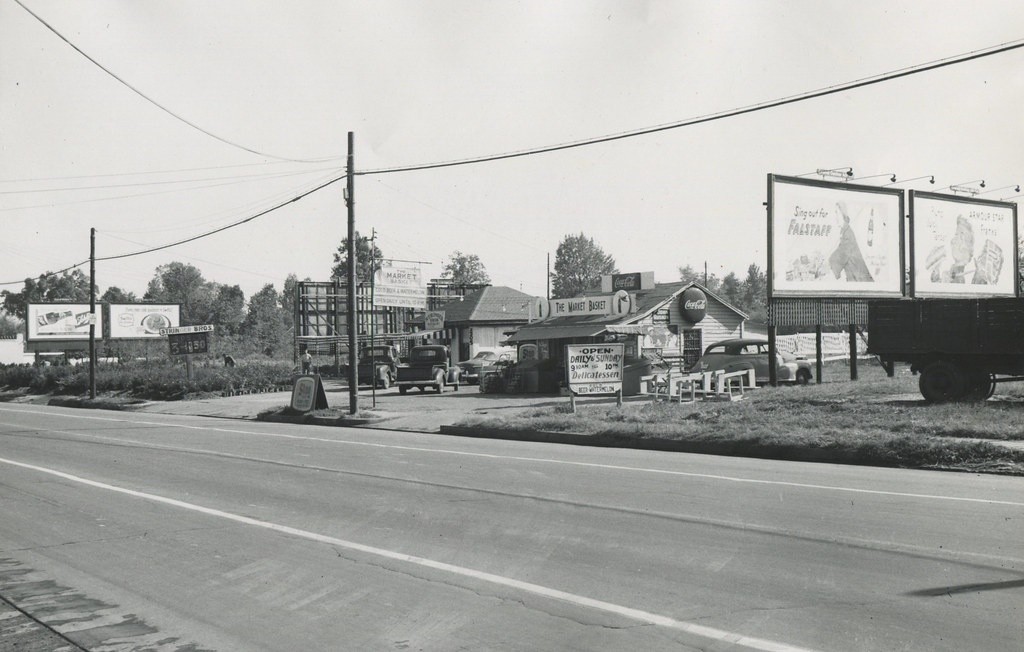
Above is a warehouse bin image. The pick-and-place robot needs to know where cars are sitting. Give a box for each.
[456,350,517,385]
[686,339,813,389]
[393,346,460,394]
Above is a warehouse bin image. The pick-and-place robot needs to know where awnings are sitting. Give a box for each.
[404,326,460,340]
[500,325,606,339]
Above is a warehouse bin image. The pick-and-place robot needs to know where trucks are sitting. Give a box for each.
[341,346,397,390]
[866,297,1024,404]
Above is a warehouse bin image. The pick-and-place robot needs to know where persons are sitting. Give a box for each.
[222,353,236,369]
[301,350,313,376]
